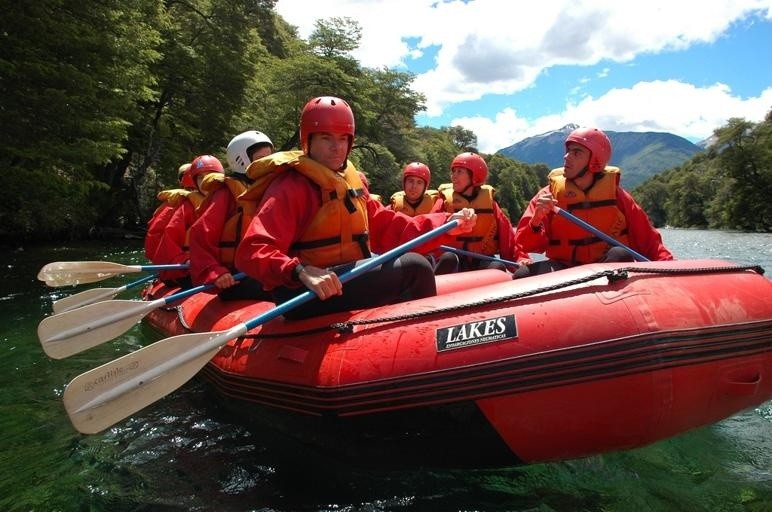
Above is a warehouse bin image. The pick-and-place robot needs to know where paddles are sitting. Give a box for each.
[37,262,188,287]
[52,274,159,314]
[64,220,467,433]
[38,271,247,359]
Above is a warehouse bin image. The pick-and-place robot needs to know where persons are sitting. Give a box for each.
[143,167,198,288]
[513,127,675,282]
[427,151,535,277]
[383,161,441,216]
[230,94,479,321]
[184,130,277,303]
[152,154,226,291]
[176,163,193,190]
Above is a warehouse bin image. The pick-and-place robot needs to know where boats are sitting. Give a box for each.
[137,259,772,464]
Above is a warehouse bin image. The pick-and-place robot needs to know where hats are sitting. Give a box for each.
[227,131,274,174]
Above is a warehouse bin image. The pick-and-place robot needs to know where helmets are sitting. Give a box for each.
[191,154,226,177]
[360,172,368,189]
[565,127,612,173]
[451,152,487,187]
[181,165,197,190]
[301,97,355,156]
[403,162,431,193]
